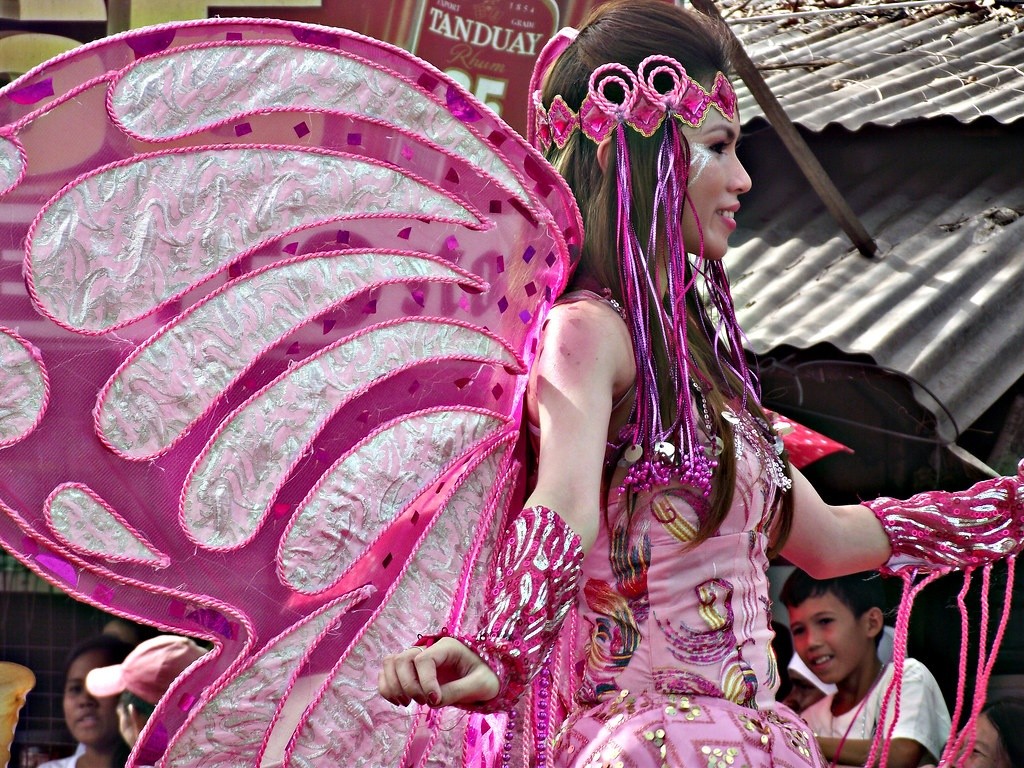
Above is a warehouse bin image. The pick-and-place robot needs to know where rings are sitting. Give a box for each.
[409,646,425,654]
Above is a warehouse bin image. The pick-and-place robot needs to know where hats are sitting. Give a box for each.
[85,635,208,707]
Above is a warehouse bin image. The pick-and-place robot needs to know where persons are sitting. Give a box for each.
[21,616,210,768]
[770,566,1024,768]
[378,0,1024,767]
